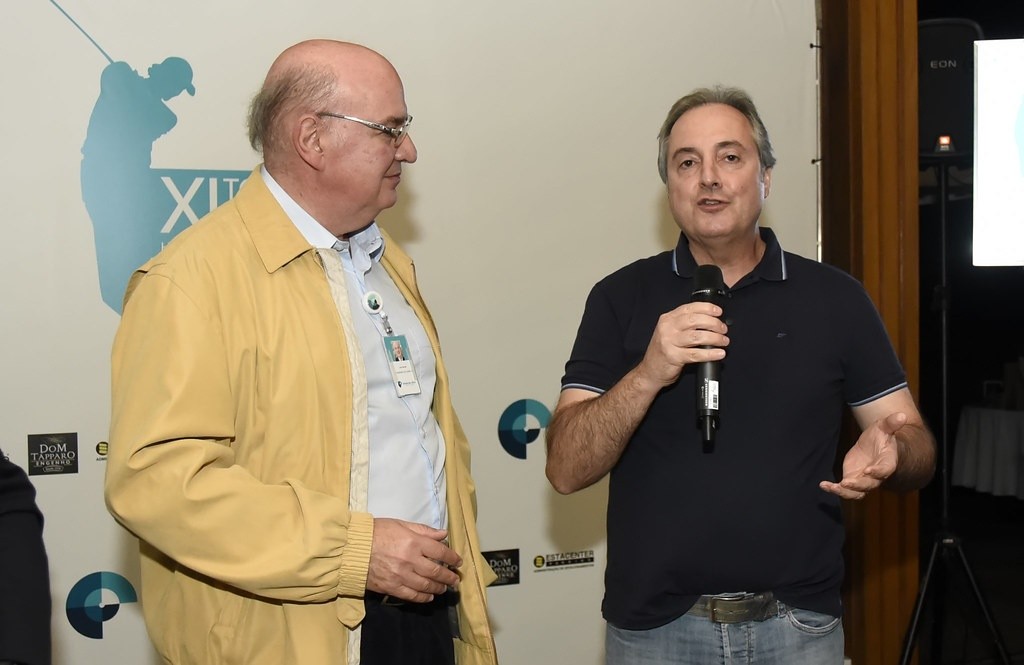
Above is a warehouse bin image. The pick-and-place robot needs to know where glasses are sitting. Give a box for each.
[313,112,411,149]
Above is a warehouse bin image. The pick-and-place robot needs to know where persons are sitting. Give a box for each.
[105,39,500,665]
[544,88,937,665]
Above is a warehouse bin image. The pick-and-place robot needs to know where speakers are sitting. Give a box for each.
[917,17,986,171]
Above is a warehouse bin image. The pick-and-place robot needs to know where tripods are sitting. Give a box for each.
[898,162,1011,665]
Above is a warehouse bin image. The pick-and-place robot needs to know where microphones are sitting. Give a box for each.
[690,264,726,441]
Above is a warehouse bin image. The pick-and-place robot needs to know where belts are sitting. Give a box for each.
[365,584,460,606]
[687,592,795,625]
